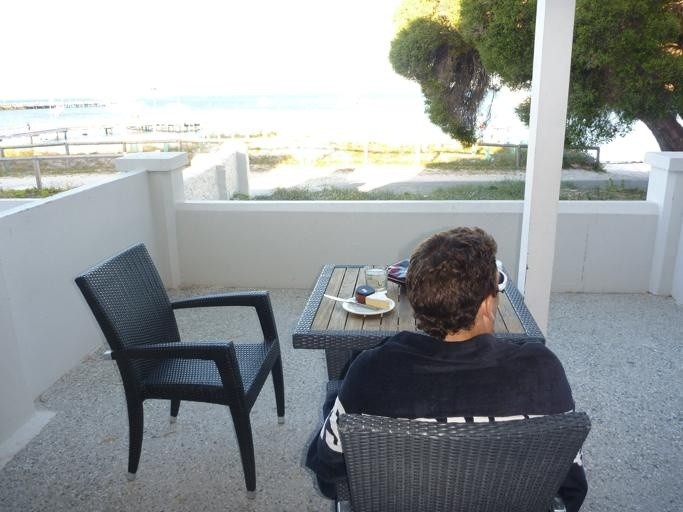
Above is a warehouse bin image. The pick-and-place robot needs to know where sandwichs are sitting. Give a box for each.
[365,291,392,310]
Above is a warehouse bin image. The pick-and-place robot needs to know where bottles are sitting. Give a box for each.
[355,286,374,303]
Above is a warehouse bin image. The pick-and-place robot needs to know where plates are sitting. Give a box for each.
[341,296,394,316]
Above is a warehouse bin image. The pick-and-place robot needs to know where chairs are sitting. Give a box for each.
[335,412,592,511]
[73,241,287,501]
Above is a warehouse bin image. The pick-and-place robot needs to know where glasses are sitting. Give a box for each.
[498,270,507,294]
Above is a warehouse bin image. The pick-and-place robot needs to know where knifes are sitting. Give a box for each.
[322,294,378,310]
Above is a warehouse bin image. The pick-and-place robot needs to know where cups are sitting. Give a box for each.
[363,265,389,295]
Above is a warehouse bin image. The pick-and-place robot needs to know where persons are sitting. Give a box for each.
[305,224,588,511]
